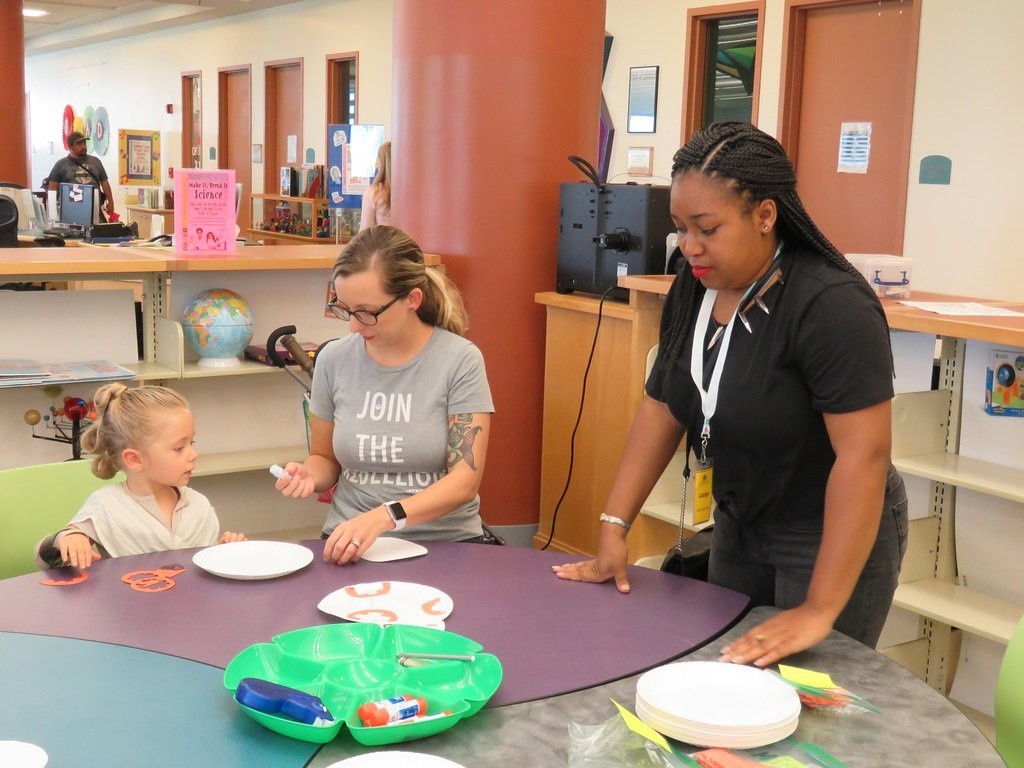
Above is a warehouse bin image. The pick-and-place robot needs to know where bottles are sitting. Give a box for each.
[163,167,174,210]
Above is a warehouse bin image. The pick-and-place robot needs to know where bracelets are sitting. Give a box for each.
[600,513,633,531]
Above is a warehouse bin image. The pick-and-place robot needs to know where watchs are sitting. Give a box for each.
[384,501,407,532]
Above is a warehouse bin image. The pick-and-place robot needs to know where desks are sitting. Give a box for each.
[0,524,1008,768]
[126,205,173,238]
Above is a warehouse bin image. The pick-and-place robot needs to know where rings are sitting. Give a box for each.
[351,540,360,548]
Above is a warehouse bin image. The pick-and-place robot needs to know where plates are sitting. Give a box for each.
[361,536,429,563]
[634,661,802,750]
[192,540,314,581]
[317,581,454,627]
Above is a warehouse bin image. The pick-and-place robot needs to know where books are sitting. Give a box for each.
[0,357,136,388]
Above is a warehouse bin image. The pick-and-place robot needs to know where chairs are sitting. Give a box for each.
[995,615,1024,768]
[0,458,128,579]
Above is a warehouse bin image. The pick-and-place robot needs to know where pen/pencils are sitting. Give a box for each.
[396,652,476,662]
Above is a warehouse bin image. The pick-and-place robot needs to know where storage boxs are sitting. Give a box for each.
[845,253,912,298]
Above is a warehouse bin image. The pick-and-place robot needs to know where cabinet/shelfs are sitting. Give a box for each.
[877,288,1024,721]
[0,244,441,538]
[246,194,350,244]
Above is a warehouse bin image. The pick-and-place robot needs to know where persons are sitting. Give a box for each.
[48,132,115,214]
[275,225,496,565]
[361,142,391,230]
[195,228,217,250]
[35,382,247,569]
[553,122,908,665]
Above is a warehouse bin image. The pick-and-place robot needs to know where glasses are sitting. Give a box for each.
[327,296,400,326]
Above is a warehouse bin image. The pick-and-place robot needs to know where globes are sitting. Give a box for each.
[181,288,256,369]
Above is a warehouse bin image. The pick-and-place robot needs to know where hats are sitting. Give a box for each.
[67,131,90,147]
